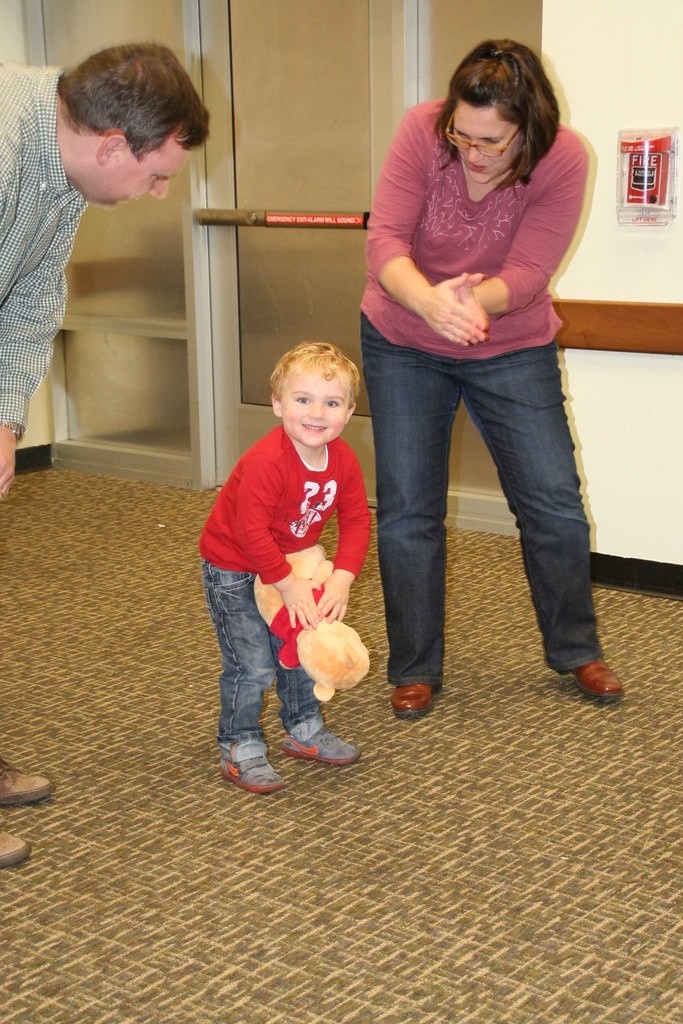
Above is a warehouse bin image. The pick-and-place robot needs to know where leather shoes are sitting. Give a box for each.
[391,684,441,721]
[571,658,625,701]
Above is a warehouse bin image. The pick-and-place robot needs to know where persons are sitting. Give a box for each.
[198,341,369,798]
[356,39,628,721]
[1,41,212,865]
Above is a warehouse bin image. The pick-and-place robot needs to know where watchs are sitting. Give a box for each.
[2,419,26,443]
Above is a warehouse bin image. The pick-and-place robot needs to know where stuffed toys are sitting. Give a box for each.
[252,546,368,704]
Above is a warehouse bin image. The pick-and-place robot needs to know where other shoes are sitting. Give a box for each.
[1,759,55,866]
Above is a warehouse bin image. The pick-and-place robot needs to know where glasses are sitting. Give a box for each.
[445,107,523,158]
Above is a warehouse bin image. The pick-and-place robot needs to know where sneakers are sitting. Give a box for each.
[217,739,286,792]
[282,713,361,767]
